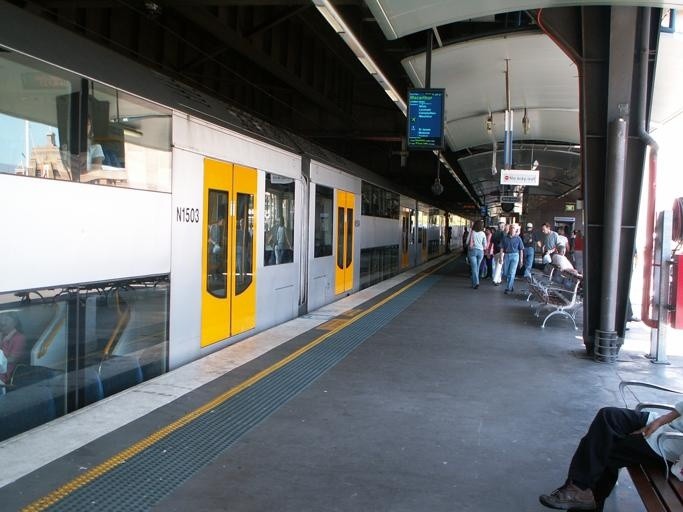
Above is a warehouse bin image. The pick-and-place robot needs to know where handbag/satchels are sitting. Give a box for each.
[467,229,474,249]
[480,257,487,278]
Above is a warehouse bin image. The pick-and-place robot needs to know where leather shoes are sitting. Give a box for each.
[540,482,596,510]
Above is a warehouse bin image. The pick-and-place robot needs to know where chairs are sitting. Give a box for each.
[523,262,583,330]
[0,338,143,443]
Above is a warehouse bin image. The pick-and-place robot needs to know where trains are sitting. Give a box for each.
[0,0,473,442]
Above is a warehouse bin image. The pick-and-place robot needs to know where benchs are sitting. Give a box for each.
[616,377,683,512]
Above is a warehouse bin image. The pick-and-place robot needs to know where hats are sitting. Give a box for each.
[527,223,532,227]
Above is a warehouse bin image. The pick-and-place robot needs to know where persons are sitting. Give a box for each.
[204,209,327,290]
[462,218,585,290]
[538,399,683,512]
[59,112,106,183]
[0,309,31,388]
